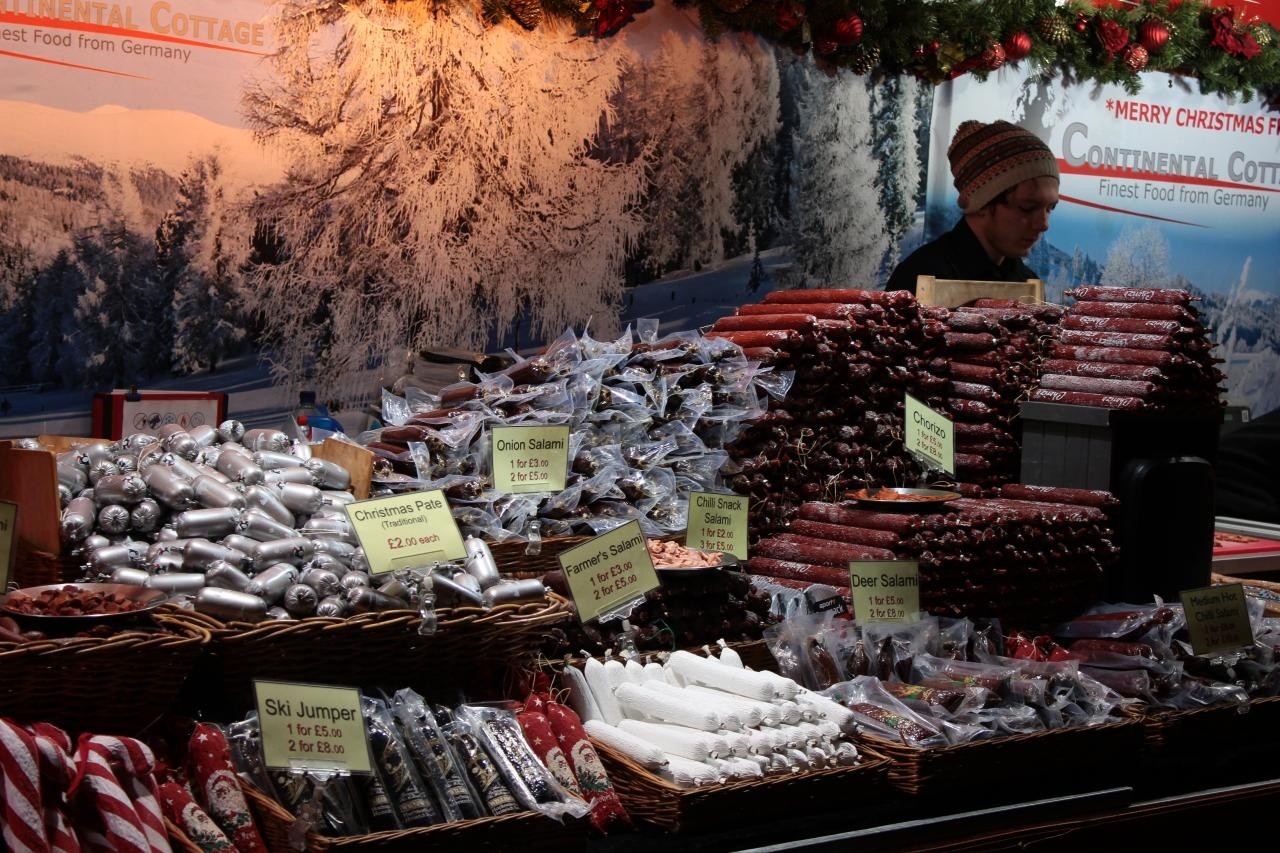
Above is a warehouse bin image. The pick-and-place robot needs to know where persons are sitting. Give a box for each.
[884,120,1059,298]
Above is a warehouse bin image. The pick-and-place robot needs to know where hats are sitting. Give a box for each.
[947,120,1059,215]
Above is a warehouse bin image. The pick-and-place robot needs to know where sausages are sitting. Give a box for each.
[0,332,859,853]
[698,282,1280,742]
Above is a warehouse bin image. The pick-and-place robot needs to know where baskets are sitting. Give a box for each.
[0,533,1280,853]
